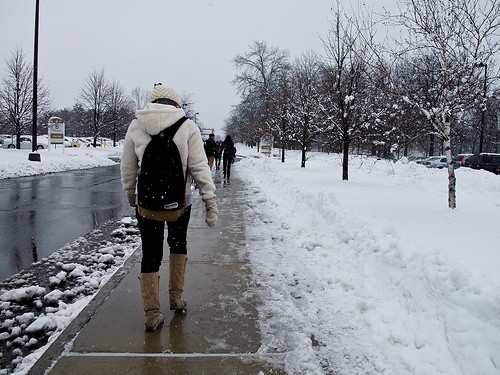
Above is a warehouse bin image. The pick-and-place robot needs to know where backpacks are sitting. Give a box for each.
[137,117,183,222]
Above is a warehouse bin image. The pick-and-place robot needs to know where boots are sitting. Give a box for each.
[168,252,186,310]
[140,272,164,332]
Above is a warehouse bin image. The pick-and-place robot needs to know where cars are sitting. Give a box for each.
[65,138,101,148]
[407,153,500,176]
[0,135,49,149]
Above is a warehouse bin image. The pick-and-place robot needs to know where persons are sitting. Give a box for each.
[219,135,236,179]
[120,85,218,330]
[204,134,224,170]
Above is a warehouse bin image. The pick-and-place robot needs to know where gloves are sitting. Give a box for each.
[126,187,137,207]
[205,199,219,228]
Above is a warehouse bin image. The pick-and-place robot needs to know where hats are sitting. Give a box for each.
[150,86,182,108]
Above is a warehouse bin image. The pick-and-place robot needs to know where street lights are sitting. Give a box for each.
[195,112,199,125]
[14,88,21,149]
[477,62,488,152]
